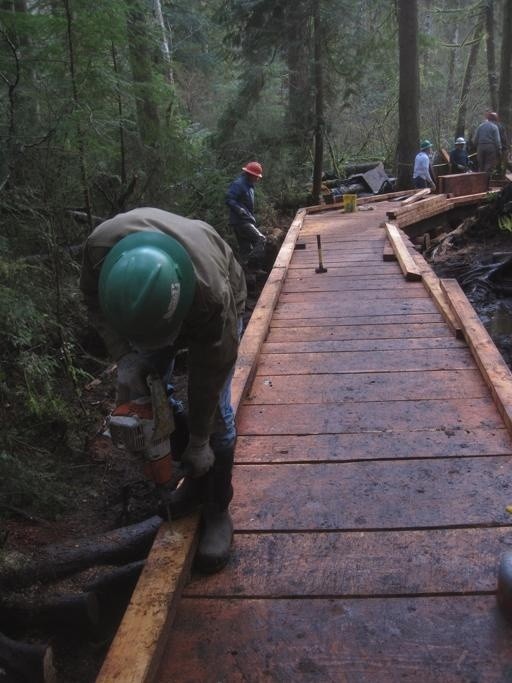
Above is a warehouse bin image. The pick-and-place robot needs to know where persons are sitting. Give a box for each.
[450,134,471,175]
[471,111,502,193]
[226,162,265,290]
[78,204,244,577]
[411,131,435,199]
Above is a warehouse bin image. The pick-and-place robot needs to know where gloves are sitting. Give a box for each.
[110,354,164,401]
[178,435,217,481]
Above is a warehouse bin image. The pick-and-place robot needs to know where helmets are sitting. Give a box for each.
[420,140,433,151]
[454,137,466,145]
[241,160,263,179]
[485,111,499,121]
[95,230,197,352]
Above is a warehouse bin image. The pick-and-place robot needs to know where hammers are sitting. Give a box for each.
[315,235,327,274]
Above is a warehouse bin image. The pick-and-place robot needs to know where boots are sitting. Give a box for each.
[189,430,239,576]
[241,259,268,297]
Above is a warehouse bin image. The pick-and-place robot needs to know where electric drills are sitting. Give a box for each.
[108,372,185,536]
